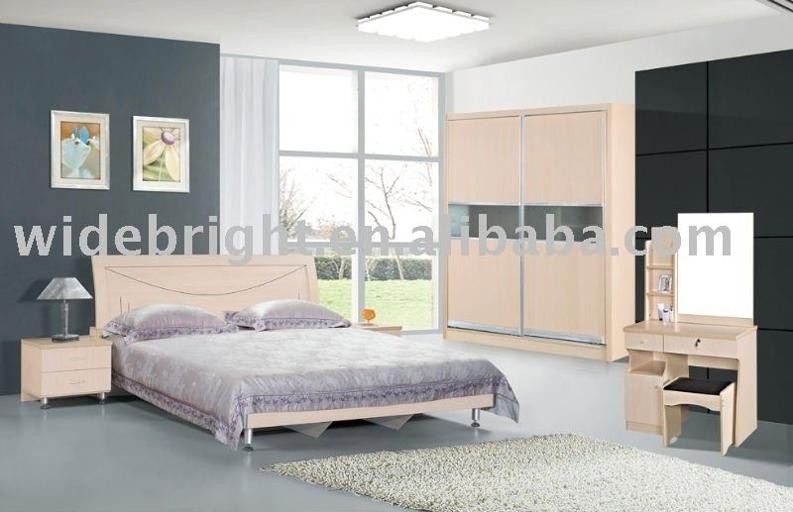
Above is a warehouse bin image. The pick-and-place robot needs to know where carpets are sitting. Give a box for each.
[258,432,793,512]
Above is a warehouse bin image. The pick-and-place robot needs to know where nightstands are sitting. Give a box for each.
[353,323,402,336]
[20,338,113,410]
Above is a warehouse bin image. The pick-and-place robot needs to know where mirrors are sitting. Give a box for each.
[676,212,755,318]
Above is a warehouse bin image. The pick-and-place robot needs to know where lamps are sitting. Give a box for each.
[357,1,491,44]
[36,276,94,342]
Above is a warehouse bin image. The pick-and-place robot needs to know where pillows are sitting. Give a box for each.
[224,299,352,331]
[103,302,240,344]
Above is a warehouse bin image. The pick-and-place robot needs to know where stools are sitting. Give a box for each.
[662,375,735,457]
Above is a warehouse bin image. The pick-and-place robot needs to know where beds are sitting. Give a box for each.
[91,254,521,452]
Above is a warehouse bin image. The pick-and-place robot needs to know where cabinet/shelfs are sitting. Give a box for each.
[623,319,759,448]
[442,103,637,365]
[644,226,675,320]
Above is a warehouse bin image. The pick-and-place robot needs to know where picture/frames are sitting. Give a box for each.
[49,110,110,191]
[132,115,190,194]
